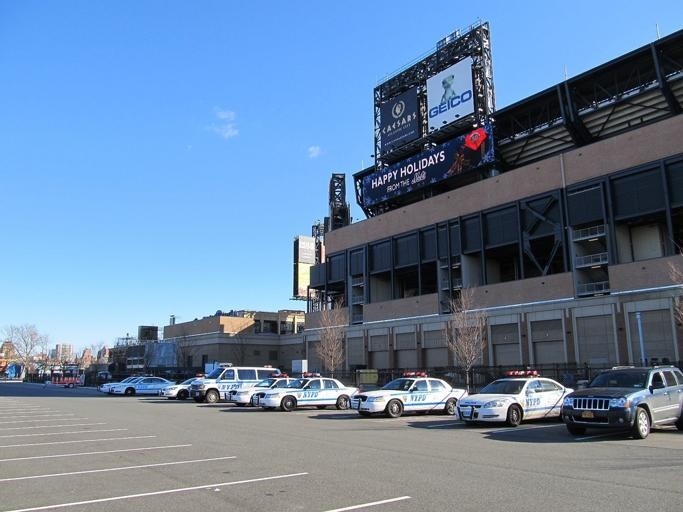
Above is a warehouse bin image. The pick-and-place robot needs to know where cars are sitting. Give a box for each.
[352,371,468,423]
[456,369,574,426]
[97,376,176,395]
[237,373,359,411]
[162,374,208,399]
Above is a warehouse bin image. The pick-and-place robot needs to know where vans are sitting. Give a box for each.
[189,361,281,403]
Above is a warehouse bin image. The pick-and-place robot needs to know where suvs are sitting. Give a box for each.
[560,366,683,439]
